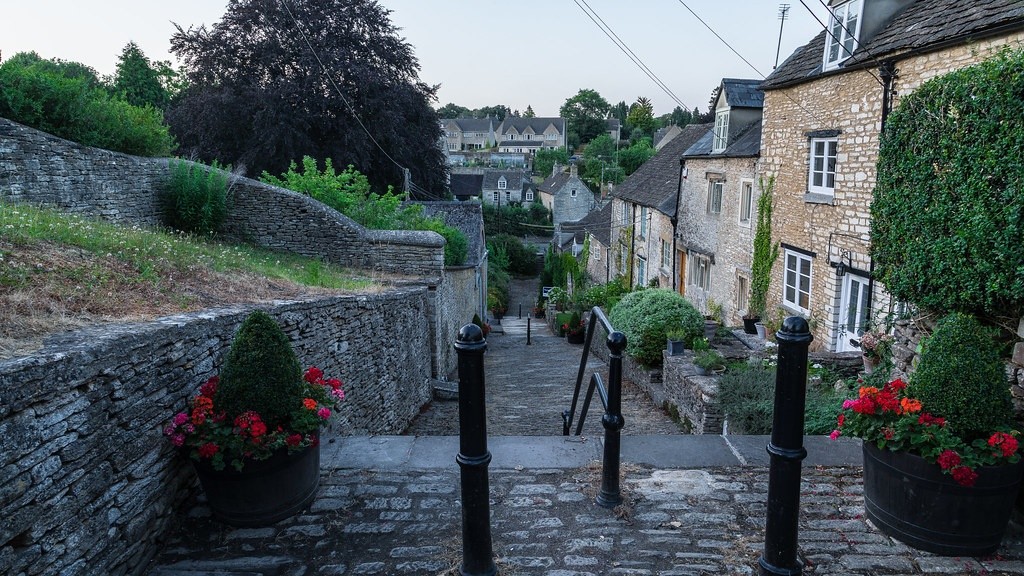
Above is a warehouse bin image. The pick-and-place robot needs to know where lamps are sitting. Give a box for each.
[835,248,853,276]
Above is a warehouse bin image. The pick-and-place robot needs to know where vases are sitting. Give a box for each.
[493,312,504,319]
[862,441,1024,559]
[192,437,321,528]
[565,332,585,344]
[535,313,544,319]
[862,353,880,375]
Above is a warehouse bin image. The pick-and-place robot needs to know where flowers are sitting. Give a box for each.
[532,300,546,314]
[858,327,895,376]
[830,311,1024,487]
[492,301,505,314]
[163,307,345,474]
[562,312,586,335]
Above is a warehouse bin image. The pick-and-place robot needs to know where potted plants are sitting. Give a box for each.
[701,295,718,342]
[692,351,726,376]
[742,305,777,339]
[666,329,687,356]
